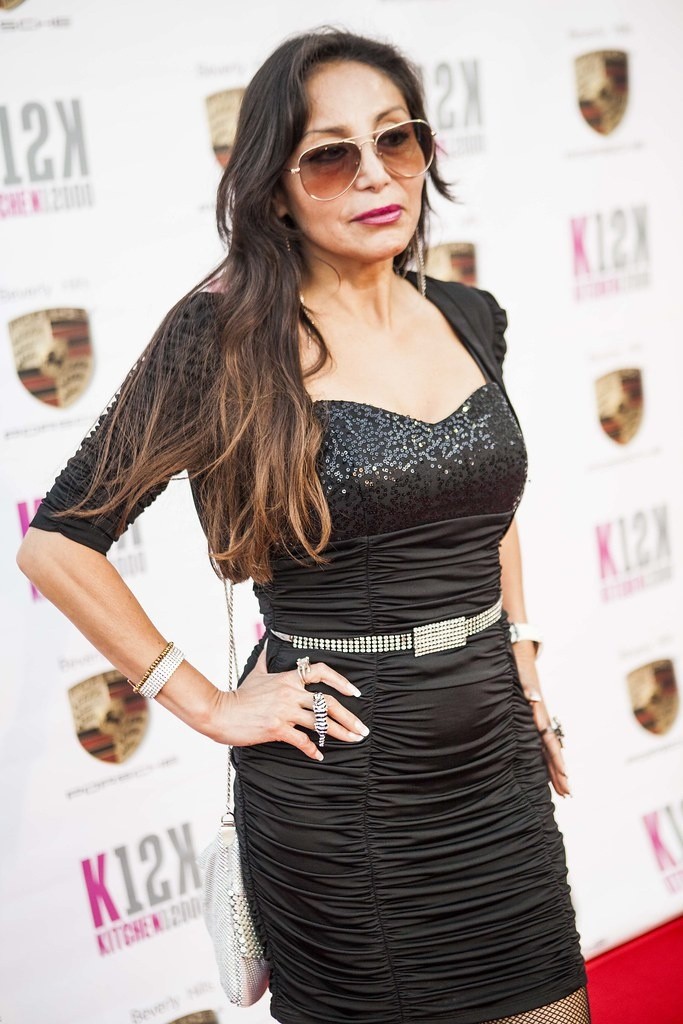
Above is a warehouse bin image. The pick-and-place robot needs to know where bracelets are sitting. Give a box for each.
[127,642,186,700]
[508,619,543,659]
[523,690,542,703]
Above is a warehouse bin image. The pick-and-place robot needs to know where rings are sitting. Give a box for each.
[312,691,328,748]
[538,717,564,749]
[296,657,311,687]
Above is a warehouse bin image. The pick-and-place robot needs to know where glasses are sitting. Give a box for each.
[279,119,437,201]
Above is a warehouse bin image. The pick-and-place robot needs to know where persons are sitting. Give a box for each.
[17,25,586,1024]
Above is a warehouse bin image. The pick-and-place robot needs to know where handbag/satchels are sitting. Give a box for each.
[201,821,270,1007]
